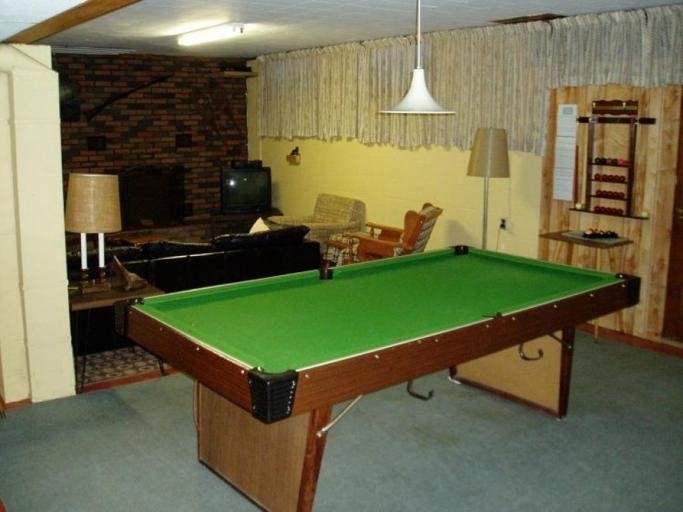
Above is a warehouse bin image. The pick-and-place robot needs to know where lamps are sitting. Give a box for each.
[65,170,123,279]
[377,0,457,116]
[466,128,514,248]
[177,22,245,46]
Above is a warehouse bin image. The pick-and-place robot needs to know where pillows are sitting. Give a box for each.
[68,245,142,261]
[143,240,215,256]
[217,226,312,243]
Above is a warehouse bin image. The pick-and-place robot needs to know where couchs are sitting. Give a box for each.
[65,223,326,290]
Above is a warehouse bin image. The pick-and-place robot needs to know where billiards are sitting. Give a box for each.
[596,190,624,199]
[594,174,626,182]
[586,227,612,235]
[594,206,623,215]
[575,203,582,209]
[642,211,649,219]
[595,157,629,165]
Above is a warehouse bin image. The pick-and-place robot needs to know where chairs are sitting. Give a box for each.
[267,191,364,256]
[335,201,443,263]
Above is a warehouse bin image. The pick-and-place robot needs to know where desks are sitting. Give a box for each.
[67,253,168,394]
[124,245,645,512]
[537,226,633,341]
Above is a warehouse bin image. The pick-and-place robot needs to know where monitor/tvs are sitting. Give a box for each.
[221,168,271,212]
